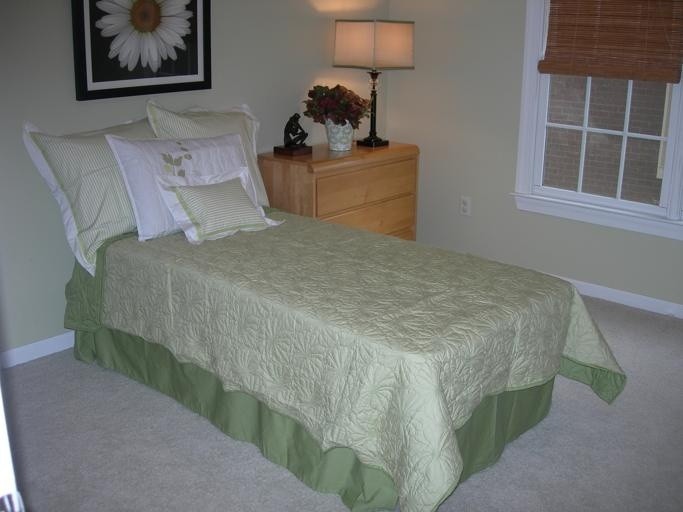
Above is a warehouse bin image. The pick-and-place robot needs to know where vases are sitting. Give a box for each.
[324,119,353,152]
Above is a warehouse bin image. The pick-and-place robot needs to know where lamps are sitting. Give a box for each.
[331,19,415,148]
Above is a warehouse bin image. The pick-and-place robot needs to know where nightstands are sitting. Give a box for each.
[256,139,419,241]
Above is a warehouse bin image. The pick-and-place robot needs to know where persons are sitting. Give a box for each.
[282,113,307,148]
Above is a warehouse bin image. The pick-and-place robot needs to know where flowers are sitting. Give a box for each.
[301,84,374,130]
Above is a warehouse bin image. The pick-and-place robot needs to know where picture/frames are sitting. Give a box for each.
[70,0,212,102]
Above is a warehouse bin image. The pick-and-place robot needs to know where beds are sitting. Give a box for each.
[74,202,572,512]
